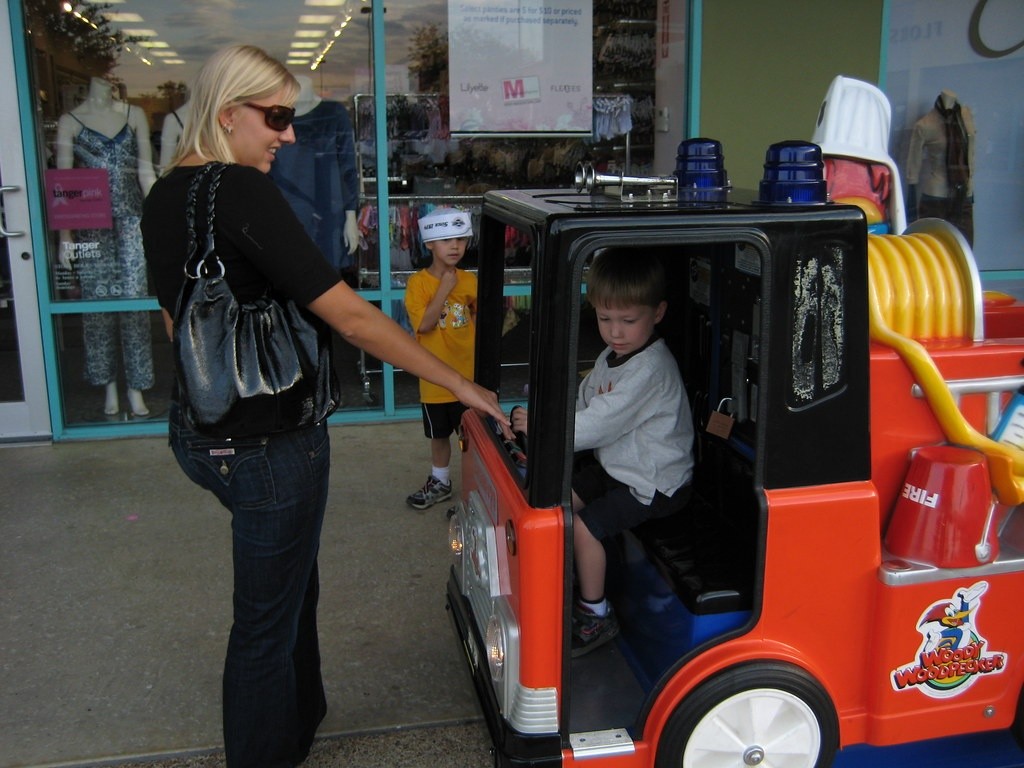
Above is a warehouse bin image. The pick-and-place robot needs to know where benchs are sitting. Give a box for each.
[615,436,760,696]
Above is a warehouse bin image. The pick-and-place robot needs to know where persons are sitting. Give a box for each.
[906,88,978,252]
[268,74,360,271]
[53,75,158,415]
[510,244,696,665]
[160,100,193,177]
[138,48,516,768]
[404,207,479,511]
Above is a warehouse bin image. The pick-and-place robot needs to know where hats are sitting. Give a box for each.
[418,207,473,245]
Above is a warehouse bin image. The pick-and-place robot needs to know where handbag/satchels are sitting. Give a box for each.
[178,160,342,443]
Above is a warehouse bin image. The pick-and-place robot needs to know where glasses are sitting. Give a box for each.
[242,101,296,131]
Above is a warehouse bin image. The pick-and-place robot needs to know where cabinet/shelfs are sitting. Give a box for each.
[353,92,630,403]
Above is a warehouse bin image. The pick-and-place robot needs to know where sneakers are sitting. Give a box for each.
[570,600,620,658]
[406,475,452,509]
